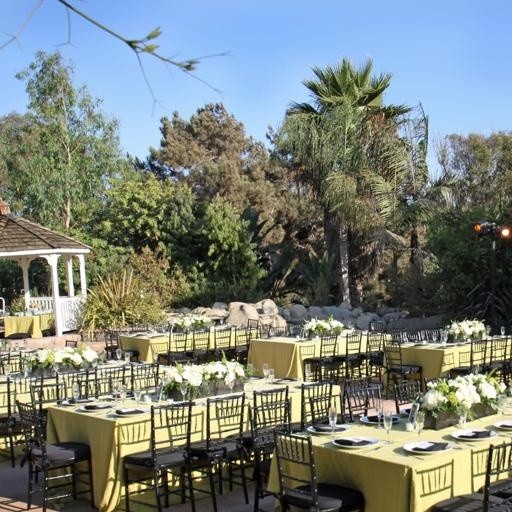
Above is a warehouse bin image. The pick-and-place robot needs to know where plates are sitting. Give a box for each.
[58,362,332,418]
[305,397,512,455]
[122,319,511,352]
[0,335,162,385]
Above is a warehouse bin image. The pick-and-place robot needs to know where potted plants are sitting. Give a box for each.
[6,294,41,316]
